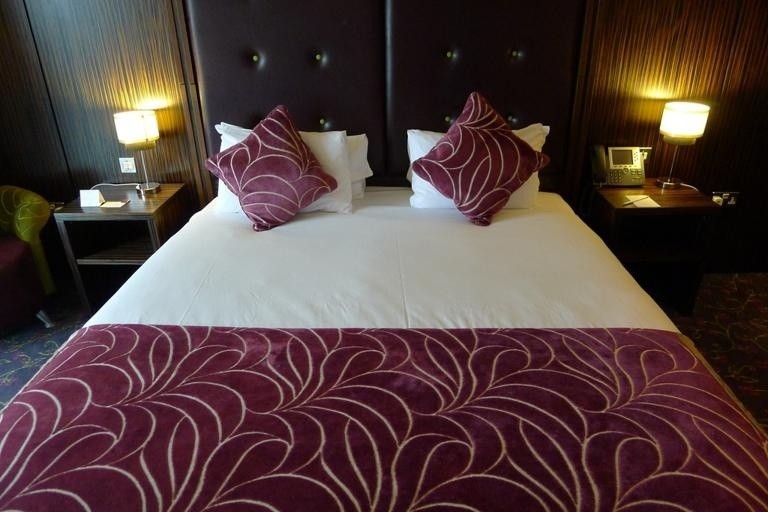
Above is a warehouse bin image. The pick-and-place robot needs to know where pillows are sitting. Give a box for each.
[411,91,551,226]
[204,104,338,231]
[406,123,551,209]
[215,121,374,216]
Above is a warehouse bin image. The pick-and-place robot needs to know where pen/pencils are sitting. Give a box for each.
[622,197,649,207]
[712,191,738,194]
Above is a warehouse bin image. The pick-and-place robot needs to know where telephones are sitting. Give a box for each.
[591,142,646,186]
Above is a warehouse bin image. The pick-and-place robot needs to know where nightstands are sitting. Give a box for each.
[594,177,721,320]
[53,181,190,318]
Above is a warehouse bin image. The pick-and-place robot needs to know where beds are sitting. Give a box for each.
[1,2,767,511]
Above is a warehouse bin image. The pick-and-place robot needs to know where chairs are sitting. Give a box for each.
[0,178,58,338]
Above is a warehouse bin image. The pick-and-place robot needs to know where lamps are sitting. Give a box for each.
[655,101,709,188]
[111,109,162,194]
